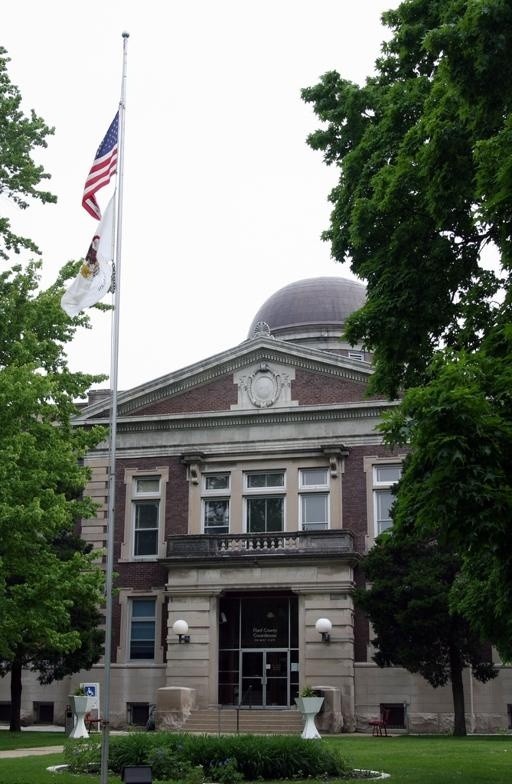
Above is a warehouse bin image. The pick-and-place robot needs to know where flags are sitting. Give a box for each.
[59,180,117,322]
[79,100,118,222]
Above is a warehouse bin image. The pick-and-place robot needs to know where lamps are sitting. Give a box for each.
[171,619,190,644]
[315,616,332,643]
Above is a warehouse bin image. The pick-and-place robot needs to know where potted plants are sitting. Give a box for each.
[294,684,326,739]
[69,684,96,739]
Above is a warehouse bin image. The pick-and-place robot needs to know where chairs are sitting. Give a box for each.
[367,709,389,737]
